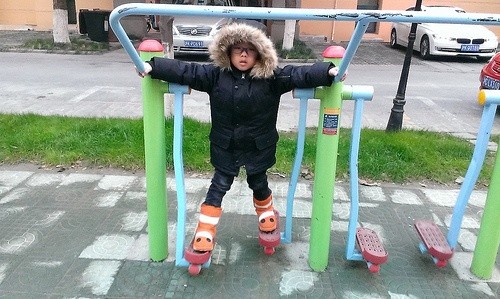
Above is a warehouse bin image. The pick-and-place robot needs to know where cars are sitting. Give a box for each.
[170,0,235,58]
[389,5,499,62]
[478,51,500,91]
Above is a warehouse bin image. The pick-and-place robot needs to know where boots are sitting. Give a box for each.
[252,193,277,232]
[192,203,223,252]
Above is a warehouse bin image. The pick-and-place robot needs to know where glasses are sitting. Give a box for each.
[230,45,259,56]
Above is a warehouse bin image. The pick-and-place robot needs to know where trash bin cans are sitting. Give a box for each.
[79,7,111,44]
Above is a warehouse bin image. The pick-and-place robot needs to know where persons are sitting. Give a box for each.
[135,18,349,253]
